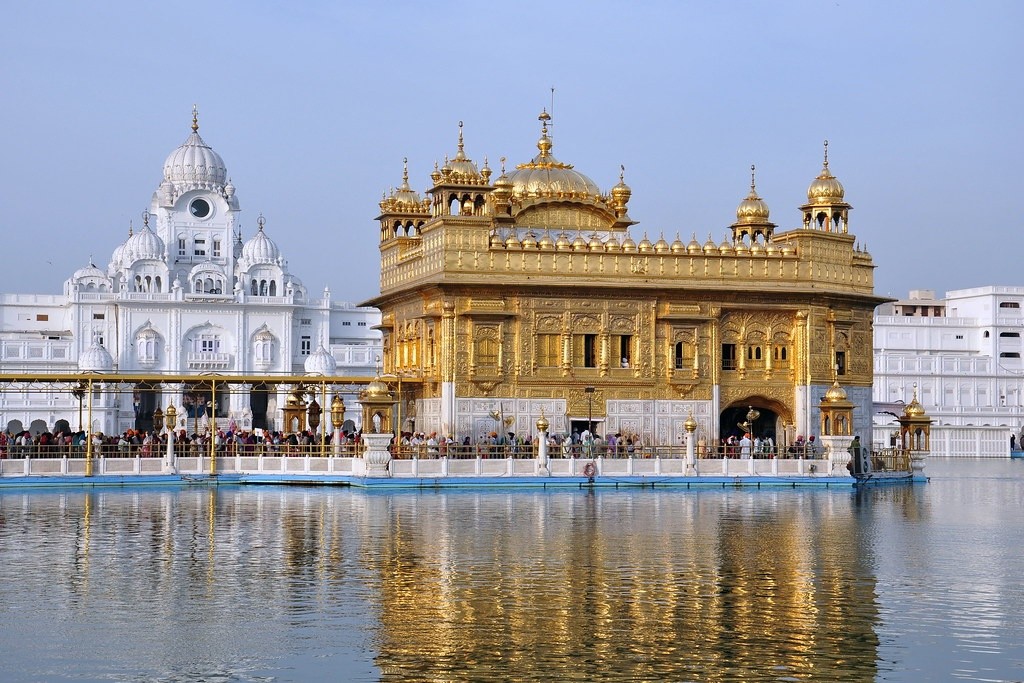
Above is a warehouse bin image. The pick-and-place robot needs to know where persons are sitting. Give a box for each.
[1020,433,1024,450]
[697,432,816,459]
[1011,434,1015,451]
[0,426,643,459]
[851,436,861,452]
